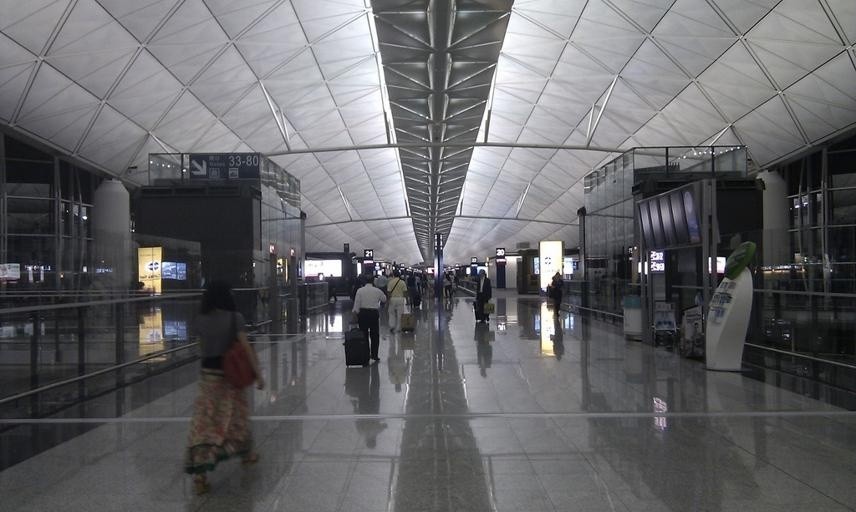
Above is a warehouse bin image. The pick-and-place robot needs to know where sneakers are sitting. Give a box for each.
[370,354,380,362]
[241,451,261,465]
[192,480,212,495]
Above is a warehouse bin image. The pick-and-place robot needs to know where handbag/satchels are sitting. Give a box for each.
[221,345,256,391]
[484,303,495,314]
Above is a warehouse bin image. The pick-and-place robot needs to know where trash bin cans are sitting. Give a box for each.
[623,296,642,341]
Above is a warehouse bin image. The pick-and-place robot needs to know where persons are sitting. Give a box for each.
[349,265,461,334]
[473,269,493,322]
[349,358,389,450]
[385,332,412,394]
[327,273,338,302]
[349,273,389,365]
[180,280,268,497]
[550,271,565,318]
[547,318,565,360]
[472,318,498,378]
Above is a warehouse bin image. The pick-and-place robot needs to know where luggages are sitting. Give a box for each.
[343,321,371,367]
[400,301,416,332]
[473,301,490,322]
